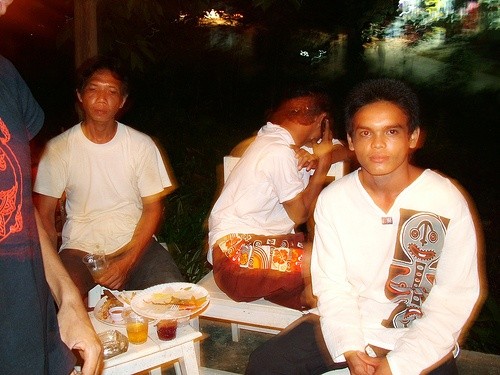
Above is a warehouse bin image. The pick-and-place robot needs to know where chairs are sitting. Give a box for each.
[197,157,344,342]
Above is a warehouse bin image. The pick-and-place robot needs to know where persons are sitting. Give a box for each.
[208,79,351,311]
[0,0,103,375]
[33,63,184,301]
[244,78,481,375]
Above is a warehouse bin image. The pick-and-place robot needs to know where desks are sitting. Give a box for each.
[75,311,202,375]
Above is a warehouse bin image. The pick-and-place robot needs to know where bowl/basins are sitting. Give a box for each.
[109,308,122,320]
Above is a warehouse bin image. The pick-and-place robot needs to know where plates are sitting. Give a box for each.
[93,292,154,328]
[131,282,210,321]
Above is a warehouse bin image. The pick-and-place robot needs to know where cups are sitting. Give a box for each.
[120,307,149,345]
[156,317,177,340]
[81,249,109,283]
